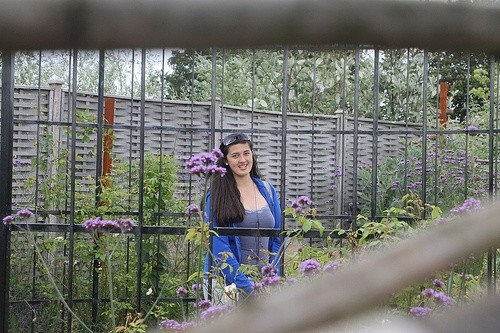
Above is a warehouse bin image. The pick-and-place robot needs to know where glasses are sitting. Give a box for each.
[222,133,250,146]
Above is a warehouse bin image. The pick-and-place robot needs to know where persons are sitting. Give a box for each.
[200,133,282,309]
